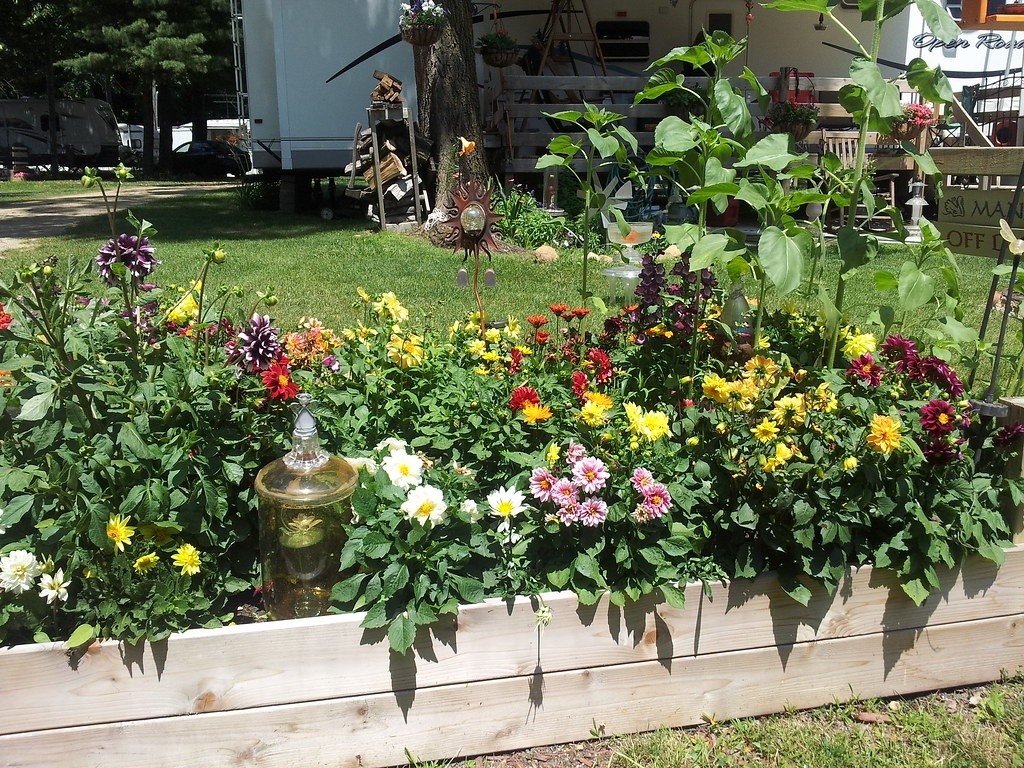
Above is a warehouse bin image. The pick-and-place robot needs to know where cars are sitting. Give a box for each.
[147,141,252,178]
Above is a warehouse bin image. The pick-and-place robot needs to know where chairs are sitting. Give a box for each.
[822,129,898,229]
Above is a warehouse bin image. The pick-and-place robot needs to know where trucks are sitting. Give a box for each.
[173,118,260,178]
[116,123,160,164]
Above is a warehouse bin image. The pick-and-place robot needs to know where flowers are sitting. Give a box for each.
[883,101,938,131]
[0,230,1024,659]
[765,98,821,128]
[396,0,452,28]
[474,29,519,50]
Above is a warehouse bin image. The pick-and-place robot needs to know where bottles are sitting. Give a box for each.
[253,409,360,620]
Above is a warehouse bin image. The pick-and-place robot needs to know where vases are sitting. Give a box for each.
[880,123,923,144]
[772,122,812,142]
[401,24,444,46]
[480,49,520,68]
[0,543,1024,768]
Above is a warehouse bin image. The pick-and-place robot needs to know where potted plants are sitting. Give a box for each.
[663,82,707,123]
